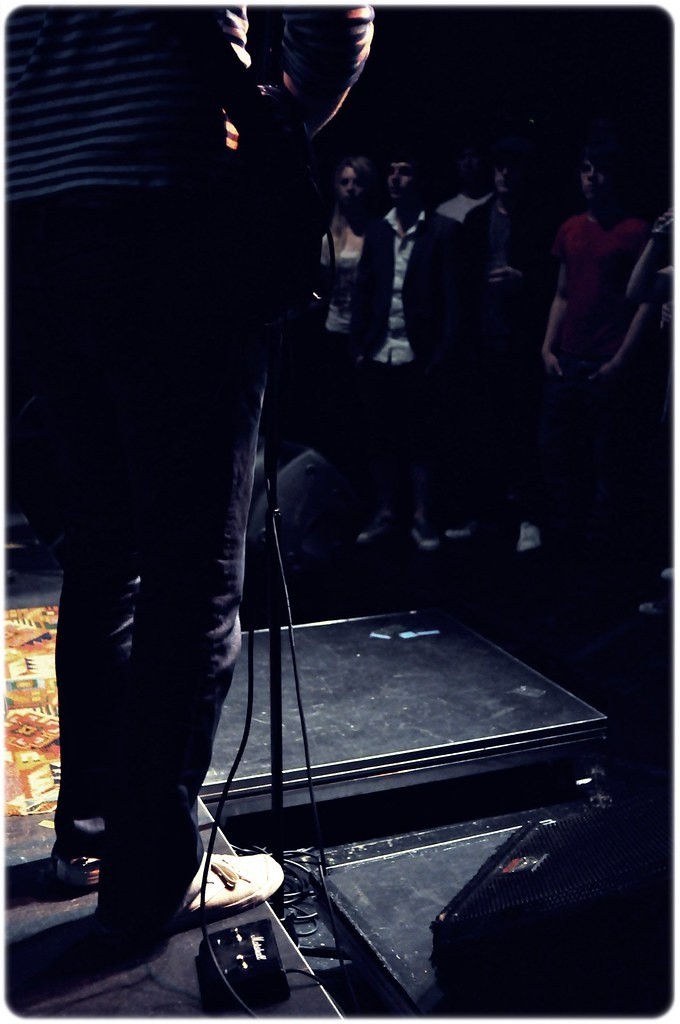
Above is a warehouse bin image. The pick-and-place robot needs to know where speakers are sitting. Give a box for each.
[431,769,675,1016]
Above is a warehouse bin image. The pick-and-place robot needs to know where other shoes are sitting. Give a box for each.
[174,852,286,919]
[46,850,101,887]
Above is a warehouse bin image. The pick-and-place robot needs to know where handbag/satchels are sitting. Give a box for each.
[279,273,331,341]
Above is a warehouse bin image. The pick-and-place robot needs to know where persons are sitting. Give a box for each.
[7,7,372,955]
[320,128,672,678]
